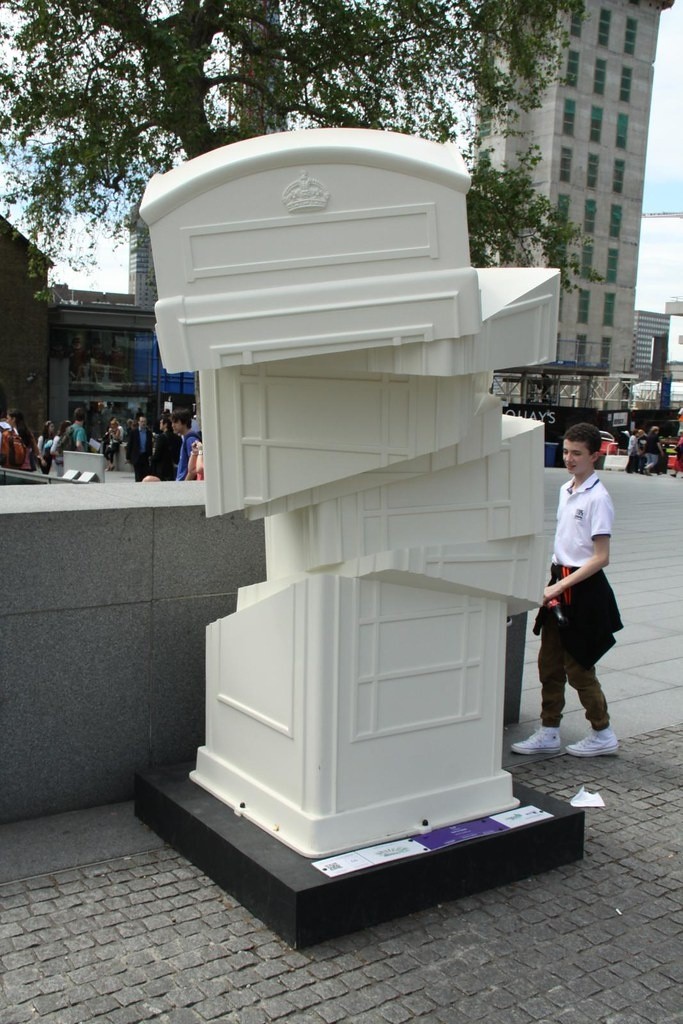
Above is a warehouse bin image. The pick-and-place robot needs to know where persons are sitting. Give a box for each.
[510,423,625,758]
[638,429,647,474]
[100,415,137,470]
[644,426,665,476]
[49,420,74,477]
[125,415,153,483]
[669,429,683,479]
[0,407,21,469]
[142,408,203,482]
[37,421,56,475]
[626,429,641,474]
[5,408,40,472]
[67,409,89,452]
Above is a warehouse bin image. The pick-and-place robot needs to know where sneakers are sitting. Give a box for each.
[565,723,619,756]
[511,725,560,754]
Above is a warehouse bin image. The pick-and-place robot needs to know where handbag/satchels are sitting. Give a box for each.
[34,435,45,465]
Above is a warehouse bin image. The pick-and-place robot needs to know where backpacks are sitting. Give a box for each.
[57,425,77,457]
[0,425,27,470]
[164,432,182,465]
[43,433,62,461]
